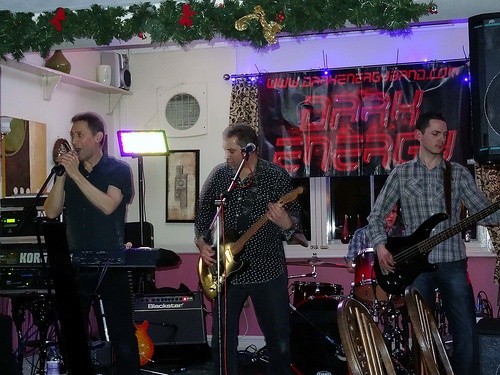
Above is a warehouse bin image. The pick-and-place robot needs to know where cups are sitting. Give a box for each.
[97,64,112,86]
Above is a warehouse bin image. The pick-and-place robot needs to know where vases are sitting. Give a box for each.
[97,64,112,84]
[45,49,71,74]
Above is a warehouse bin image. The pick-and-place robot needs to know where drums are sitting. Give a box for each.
[353,248,389,302]
[291,281,344,306]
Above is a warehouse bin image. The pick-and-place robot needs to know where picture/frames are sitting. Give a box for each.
[165,149,200,223]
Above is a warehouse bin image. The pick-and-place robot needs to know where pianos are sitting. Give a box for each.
[0,244,182,342]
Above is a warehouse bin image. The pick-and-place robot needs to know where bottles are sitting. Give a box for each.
[464,210,472,242]
[45,49,71,74]
[356,213,361,229]
[44,346,60,375]
[342,214,348,244]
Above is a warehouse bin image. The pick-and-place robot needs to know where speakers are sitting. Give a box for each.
[100,52,132,91]
[135,291,207,367]
[123,221,155,297]
[467,11,500,166]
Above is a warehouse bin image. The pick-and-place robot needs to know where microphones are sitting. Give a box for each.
[57,151,78,177]
[164,322,179,330]
[241,143,256,152]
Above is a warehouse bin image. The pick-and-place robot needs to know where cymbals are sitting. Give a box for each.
[285,256,351,269]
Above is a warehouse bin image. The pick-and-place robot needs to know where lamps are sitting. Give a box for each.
[118,129,170,248]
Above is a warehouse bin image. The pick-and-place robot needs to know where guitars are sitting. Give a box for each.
[196,185,305,301]
[373,200,500,295]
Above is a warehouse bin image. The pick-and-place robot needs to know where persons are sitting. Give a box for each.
[366,112,500,375]
[195,125,302,375]
[345,202,397,273]
[44,113,133,375]
